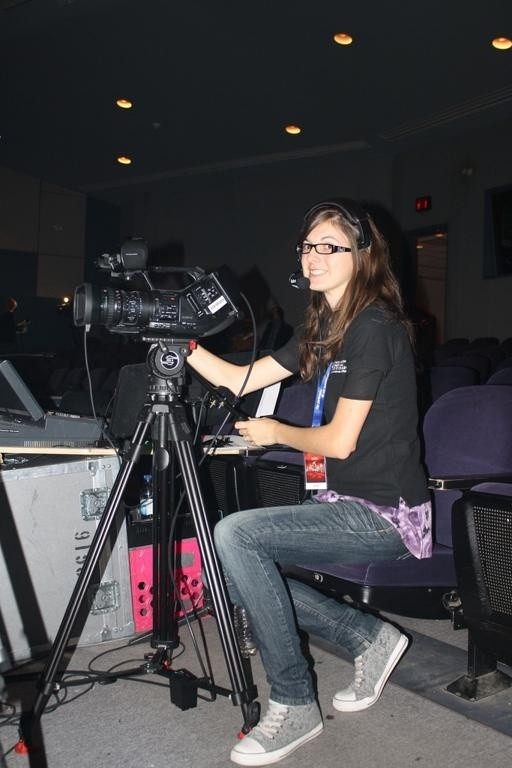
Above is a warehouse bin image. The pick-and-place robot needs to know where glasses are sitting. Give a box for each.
[294,240,355,256]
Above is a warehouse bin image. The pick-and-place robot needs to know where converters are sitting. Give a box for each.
[168,668,199,709]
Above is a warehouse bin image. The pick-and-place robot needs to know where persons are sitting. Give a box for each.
[184,194,439,768]
[0,296,293,419]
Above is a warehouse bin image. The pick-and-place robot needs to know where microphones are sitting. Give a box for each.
[287,271,311,292]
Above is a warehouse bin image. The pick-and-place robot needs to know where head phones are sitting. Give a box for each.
[294,200,372,250]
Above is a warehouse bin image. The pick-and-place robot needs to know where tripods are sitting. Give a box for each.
[15,338,262,754]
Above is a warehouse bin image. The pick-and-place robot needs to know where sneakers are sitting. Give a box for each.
[331,620,410,714]
[229,695,325,768]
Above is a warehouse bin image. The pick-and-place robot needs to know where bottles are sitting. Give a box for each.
[233,604,256,659]
[140,476,155,518]
